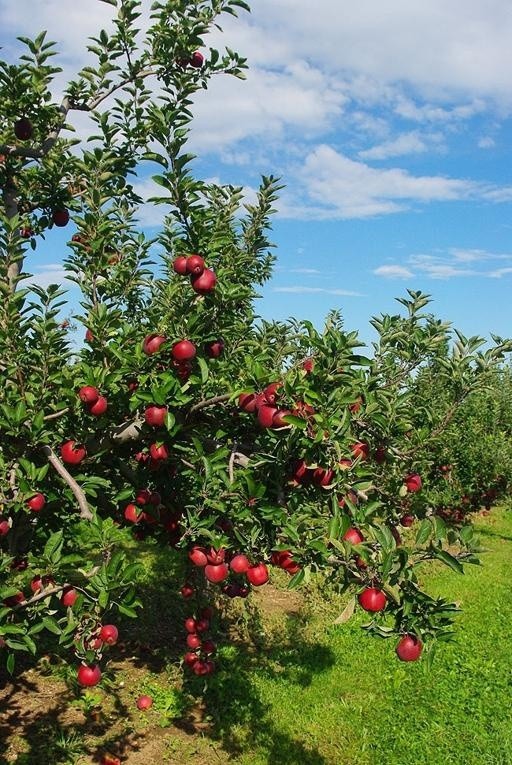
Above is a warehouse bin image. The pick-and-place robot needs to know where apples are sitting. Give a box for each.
[1,102,128,235]
[188,50,205,67]
[0,253,479,709]
